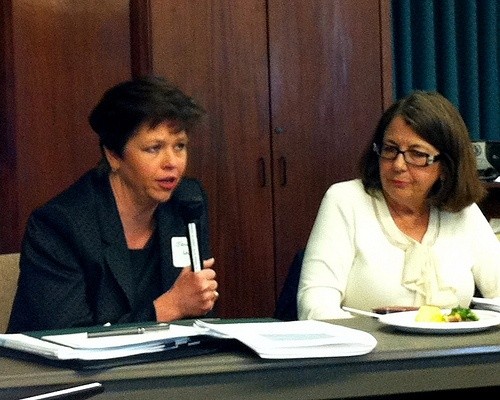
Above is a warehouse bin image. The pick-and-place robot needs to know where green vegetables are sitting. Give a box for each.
[447,304,479,321]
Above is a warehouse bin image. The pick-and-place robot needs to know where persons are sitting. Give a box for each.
[6,75,218,336]
[295,91,500,321]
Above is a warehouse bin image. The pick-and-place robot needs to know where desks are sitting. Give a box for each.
[0,316,500,400]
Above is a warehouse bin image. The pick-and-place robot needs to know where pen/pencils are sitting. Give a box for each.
[87,327,145,338]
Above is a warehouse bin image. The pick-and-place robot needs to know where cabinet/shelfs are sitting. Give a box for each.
[0,0,396,318]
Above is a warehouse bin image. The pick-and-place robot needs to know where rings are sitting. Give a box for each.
[215,292,219,299]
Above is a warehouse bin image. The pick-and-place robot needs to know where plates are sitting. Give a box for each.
[378,308,500,334]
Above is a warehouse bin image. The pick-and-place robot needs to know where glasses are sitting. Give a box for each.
[373,140,441,167]
